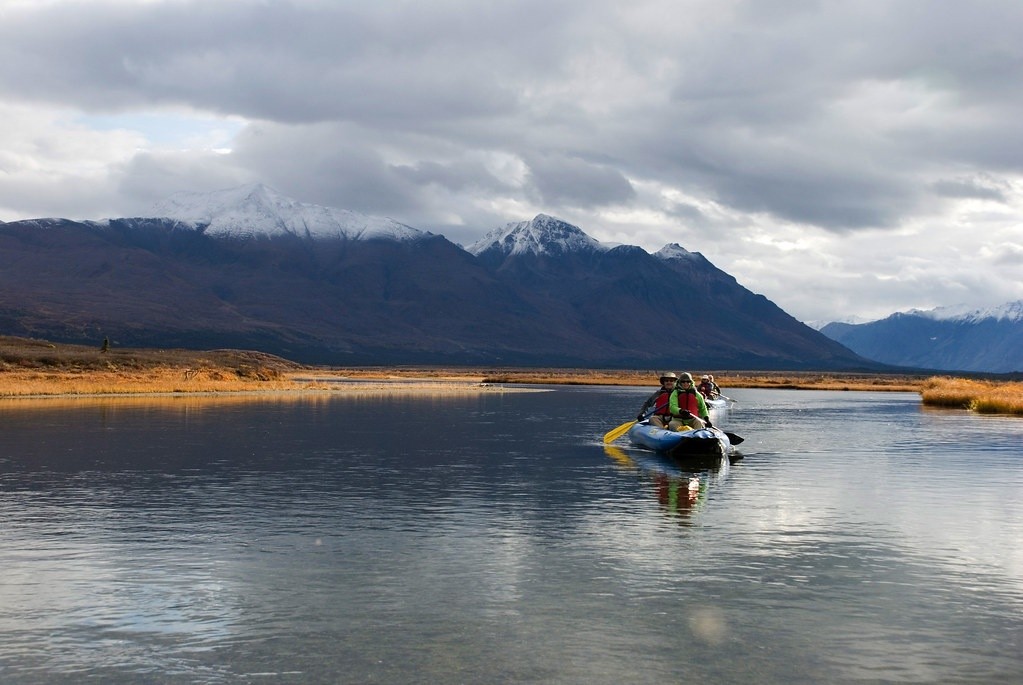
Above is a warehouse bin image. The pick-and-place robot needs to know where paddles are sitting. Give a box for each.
[682,409,745,446]
[710,390,738,403]
[603,402,669,444]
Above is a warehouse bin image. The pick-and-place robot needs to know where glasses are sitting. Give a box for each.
[681,380,691,383]
[664,379,674,382]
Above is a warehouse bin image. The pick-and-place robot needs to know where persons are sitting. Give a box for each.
[669,373,712,432]
[697,375,718,400]
[637,372,678,427]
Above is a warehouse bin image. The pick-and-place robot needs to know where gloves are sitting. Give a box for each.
[637,415,645,422]
[679,409,690,416]
[704,416,712,428]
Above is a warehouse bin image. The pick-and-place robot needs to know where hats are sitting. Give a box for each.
[702,375,708,380]
[680,372,692,380]
[660,372,678,385]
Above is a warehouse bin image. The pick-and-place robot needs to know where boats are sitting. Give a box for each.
[629,420,731,455]
[705,399,727,409]
